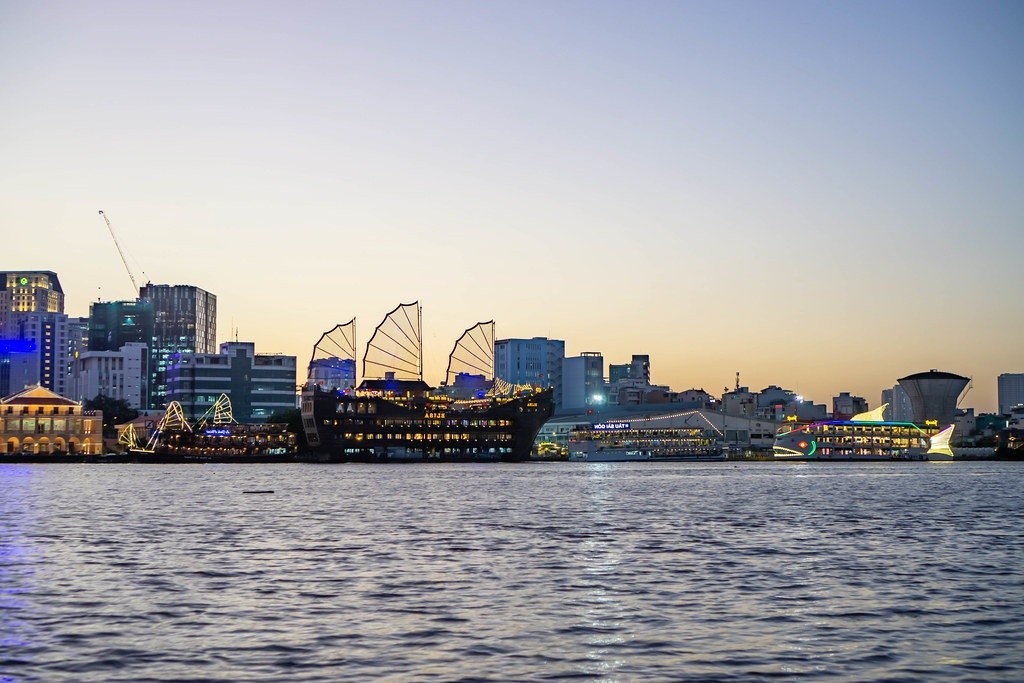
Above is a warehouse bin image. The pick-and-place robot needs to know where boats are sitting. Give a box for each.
[772,402,954,461]
[566,429,728,463]
[300,380,554,463]
[994,428,1024,462]
[152,421,300,465]
